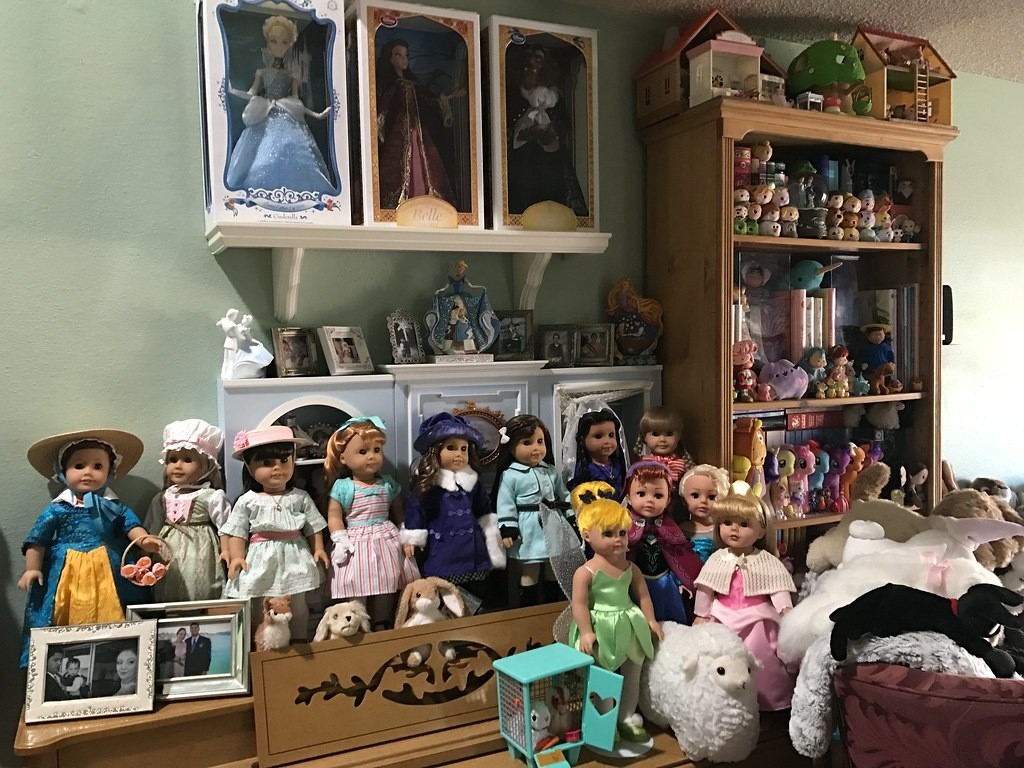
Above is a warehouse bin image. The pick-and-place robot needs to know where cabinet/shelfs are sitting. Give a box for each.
[636,94,961,559]
[217,361,664,508]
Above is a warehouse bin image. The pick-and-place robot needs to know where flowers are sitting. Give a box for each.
[233,429,248,450]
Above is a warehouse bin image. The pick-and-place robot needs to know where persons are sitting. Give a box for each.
[227,16,588,215]
[46,647,88,700]
[19,398,703,667]
[604,278,664,357]
[112,643,138,695]
[678,463,796,711]
[282,323,605,369]
[215,308,274,379]
[567,480,665,749]
[167,622,212,677]
[425,260,500,353]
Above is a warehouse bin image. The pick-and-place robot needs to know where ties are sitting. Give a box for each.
[191,639,196,653]
[54,676,59,684]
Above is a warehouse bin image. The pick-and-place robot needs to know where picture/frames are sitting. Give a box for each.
[573,322,616,367]
[318,325,375,377]
[538,323,581,361]
[126,597,252,705]
[387,306,428,366]
[24,618,158,725]
[271,326,321,378]
[492,309,535,362]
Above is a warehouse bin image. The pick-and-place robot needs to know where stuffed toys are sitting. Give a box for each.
[254,594,291,652]
[313,600,371,642]
[732,261,921,403]
[393,576,464,707]
[734,421,1024,758]
[639,622,758,761]
[507,687,573,749]
[734,140,917,242]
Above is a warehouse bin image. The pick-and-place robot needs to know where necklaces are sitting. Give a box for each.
[271,495,283,512]
[602,462,614,480]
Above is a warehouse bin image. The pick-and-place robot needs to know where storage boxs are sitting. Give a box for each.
[194,0,600,237]
